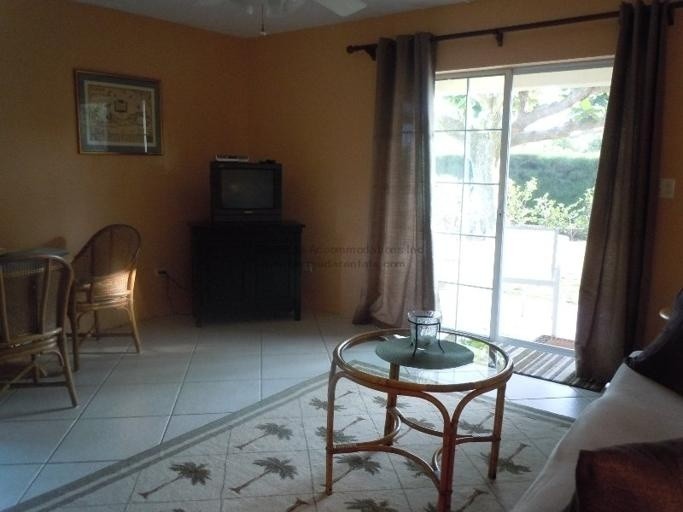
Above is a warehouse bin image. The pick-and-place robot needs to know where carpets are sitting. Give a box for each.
[490,335,615,393]
[0,358,579,511]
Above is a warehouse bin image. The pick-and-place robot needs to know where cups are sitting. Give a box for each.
[406,309,443,352]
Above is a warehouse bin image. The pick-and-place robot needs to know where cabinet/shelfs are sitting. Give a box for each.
[187,218,305,322]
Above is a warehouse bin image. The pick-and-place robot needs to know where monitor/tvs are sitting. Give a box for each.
[210,161,281,223]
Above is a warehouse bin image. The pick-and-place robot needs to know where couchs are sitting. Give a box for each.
[502,349,683,511]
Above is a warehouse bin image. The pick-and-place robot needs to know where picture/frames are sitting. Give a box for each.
[72,66,166,159]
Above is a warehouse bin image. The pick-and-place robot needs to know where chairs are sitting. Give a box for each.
[497,221,564,338]
[0,223,142,409]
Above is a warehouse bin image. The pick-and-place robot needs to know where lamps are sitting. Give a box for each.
[236,0,297,38]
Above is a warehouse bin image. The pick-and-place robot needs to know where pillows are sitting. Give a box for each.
[569,435,683,509]
[621,287,683,397]
